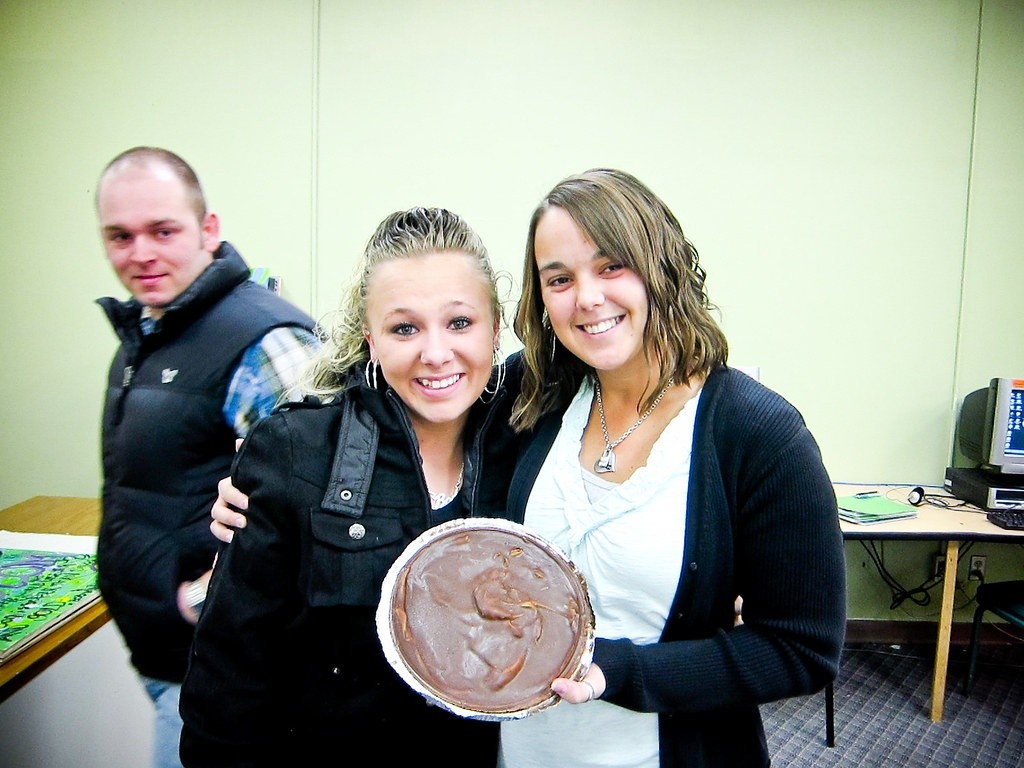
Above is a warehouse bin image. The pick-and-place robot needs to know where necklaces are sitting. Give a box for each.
[428,463,465,508]
[593,374,676,473]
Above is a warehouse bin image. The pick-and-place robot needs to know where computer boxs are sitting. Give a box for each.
[943,467,1024,511]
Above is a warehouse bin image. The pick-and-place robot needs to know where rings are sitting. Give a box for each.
[582,680,596,702]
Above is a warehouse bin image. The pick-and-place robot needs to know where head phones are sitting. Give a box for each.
[908,487,925,505]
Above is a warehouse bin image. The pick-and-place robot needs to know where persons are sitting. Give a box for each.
[173,205,512,768]
[91,147,328,768]
[208,166,849,768]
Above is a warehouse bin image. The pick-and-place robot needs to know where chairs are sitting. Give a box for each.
[957,580,1023,695]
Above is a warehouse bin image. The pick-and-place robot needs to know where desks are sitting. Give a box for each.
[824,481,1024,749]
[0,493,155,767]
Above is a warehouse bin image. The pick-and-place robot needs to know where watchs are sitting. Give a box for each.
[185,580,207,615]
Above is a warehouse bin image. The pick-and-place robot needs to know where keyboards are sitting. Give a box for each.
[987,511,1024,531]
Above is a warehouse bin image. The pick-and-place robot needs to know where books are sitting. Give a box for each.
[835,496,918,526]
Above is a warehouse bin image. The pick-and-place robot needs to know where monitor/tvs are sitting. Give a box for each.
[958,378,1024,490]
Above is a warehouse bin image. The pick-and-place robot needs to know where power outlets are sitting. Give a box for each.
[969,555,986,581]
